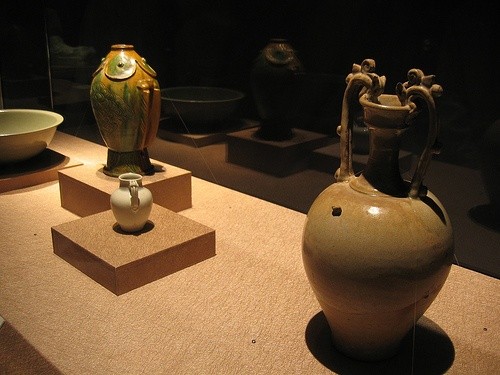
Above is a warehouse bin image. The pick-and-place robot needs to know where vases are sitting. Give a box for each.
[302,58,455,363]
[89,44,162,177]
[251,38,304,138]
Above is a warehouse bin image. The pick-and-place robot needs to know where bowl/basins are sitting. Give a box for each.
[0,109,64,163]
[159,86,245,132]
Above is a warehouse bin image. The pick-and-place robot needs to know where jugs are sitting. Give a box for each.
[110,172,153,232]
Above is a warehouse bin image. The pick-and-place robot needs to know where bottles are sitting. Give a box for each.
[302,58,455,361]
